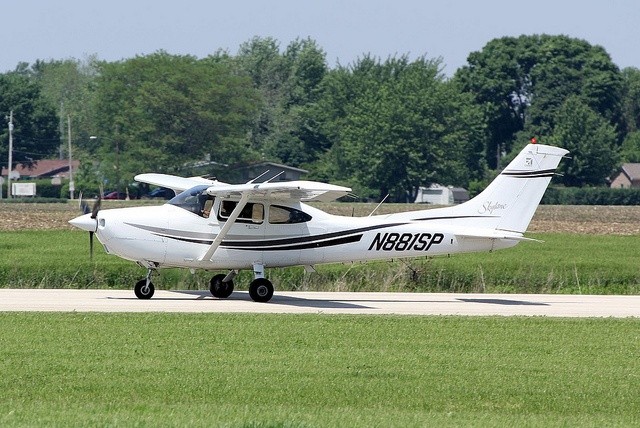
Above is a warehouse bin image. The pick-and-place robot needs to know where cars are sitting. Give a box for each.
[100,191,128,198]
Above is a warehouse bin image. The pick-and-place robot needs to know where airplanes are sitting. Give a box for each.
[65,142,569,303]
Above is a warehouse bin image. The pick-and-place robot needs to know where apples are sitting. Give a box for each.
[531,139,537,145]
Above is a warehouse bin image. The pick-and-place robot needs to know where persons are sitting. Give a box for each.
[201,200,237,219]
